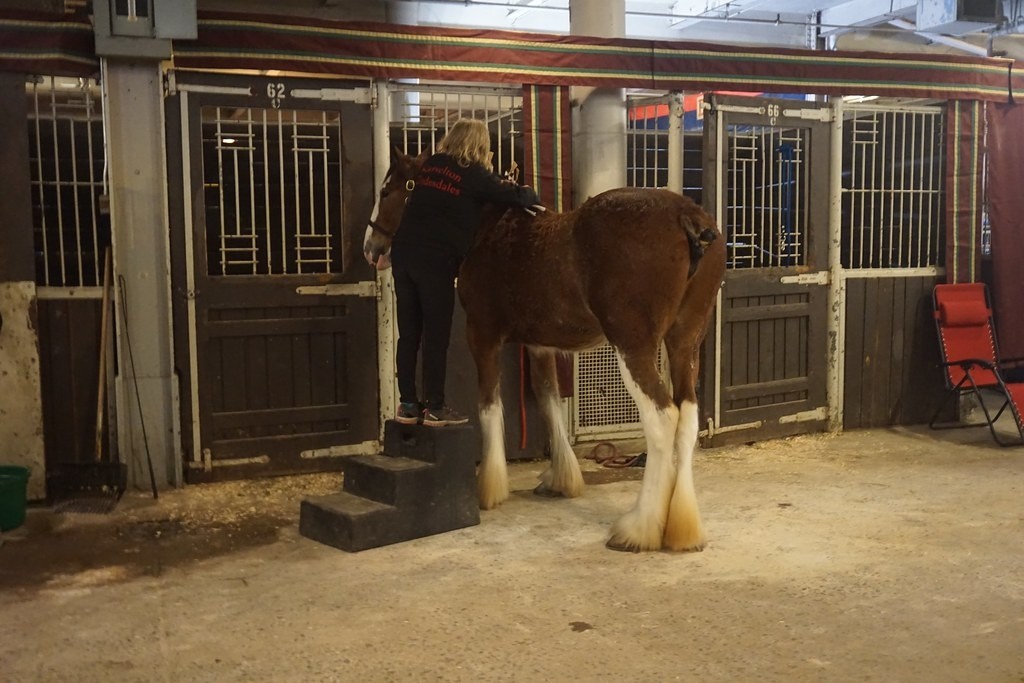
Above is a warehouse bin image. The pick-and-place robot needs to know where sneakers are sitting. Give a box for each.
[422,404,468,427]
[396,402,425,424]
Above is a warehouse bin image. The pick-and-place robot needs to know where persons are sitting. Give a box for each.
[391,118,537,426]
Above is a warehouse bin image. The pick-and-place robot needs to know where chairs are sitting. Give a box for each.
[928,283,1024,448]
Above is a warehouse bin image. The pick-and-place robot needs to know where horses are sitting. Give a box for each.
[363,140,728,554]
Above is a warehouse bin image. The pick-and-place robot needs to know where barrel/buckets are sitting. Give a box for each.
[0,463,31,532]
